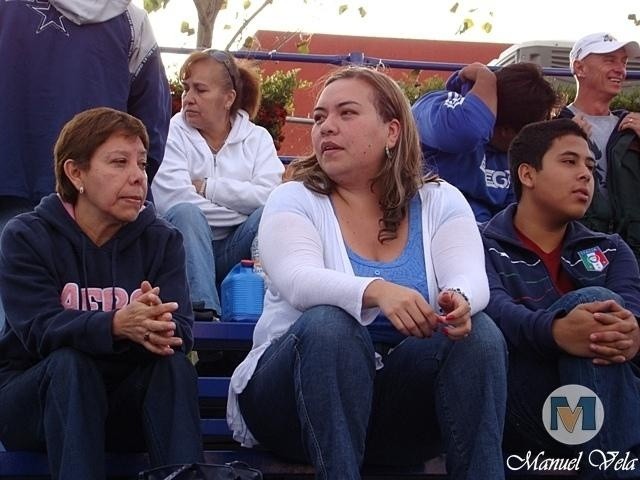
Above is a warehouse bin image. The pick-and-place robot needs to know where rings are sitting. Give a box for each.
[629,119,633,122]
[144,329,151,342]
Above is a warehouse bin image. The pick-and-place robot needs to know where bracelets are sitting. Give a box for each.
[438,285,473,314]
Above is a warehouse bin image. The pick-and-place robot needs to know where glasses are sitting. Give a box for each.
[200,48,238,91]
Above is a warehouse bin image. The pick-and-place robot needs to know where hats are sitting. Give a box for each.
[568,32,640,76]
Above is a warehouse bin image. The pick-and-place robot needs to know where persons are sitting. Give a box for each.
[1,0,173,220]
[410,59,565,232]
[556,29,640,264]
[150,44,288,320]
[0,104,209,480]
[483,114,640,480]
[223,64,514,480]
